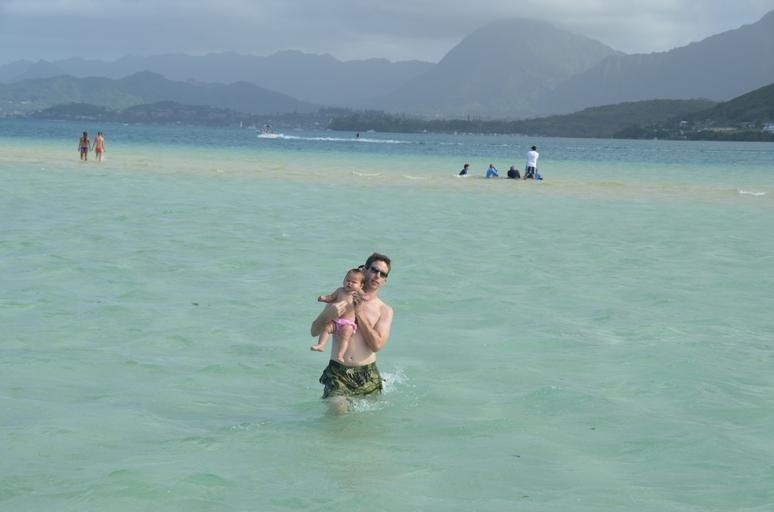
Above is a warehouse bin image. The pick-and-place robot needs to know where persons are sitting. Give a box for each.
[486,163,499,178]
[507,165,520,179]
[310,264,371,364]
[78,130,91,160]
[458,163,469,175]
[526,167,543,181]
[522,145,540,179]
[91,131,105,161]
[309,252,394,417]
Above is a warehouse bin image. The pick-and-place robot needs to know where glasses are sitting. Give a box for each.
[367,265,388,279]
